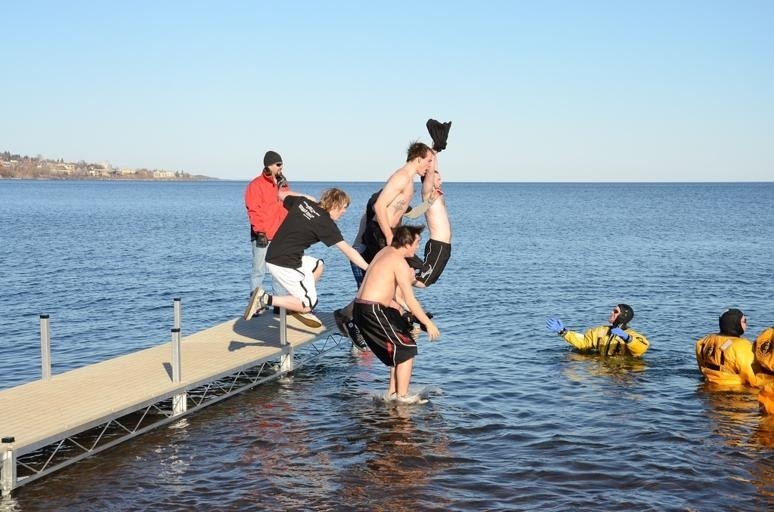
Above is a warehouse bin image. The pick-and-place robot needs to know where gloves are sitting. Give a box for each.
[257,233,269,247]
[546,317,564,333]
[611,328,631,342]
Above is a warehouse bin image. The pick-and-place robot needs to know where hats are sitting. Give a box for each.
[613,304,634,326]
[264,152,282,165]
[719,309,744,335]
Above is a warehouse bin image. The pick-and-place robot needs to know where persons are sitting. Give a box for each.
[245,151,289,319]
[354,225,439,398]
[695,309,771,391]
[244,188,370,328]
[752,328,774,388]
[332,142,452,351]
[545,303,649,359]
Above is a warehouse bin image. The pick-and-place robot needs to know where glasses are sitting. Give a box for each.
[277,164,281,166]
[613,309,619,314]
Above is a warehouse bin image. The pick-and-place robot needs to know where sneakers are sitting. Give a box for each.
[244,288,266,321]
[334,309,371,352]
[293,312,322,327]
